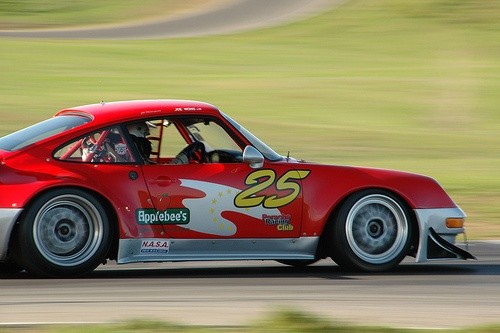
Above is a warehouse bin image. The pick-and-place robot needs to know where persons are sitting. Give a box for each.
[109,121,204,164]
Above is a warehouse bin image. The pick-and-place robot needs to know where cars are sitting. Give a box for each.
[0,100,479,278]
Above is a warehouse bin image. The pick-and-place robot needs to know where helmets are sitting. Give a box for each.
[108,122,153,140]
